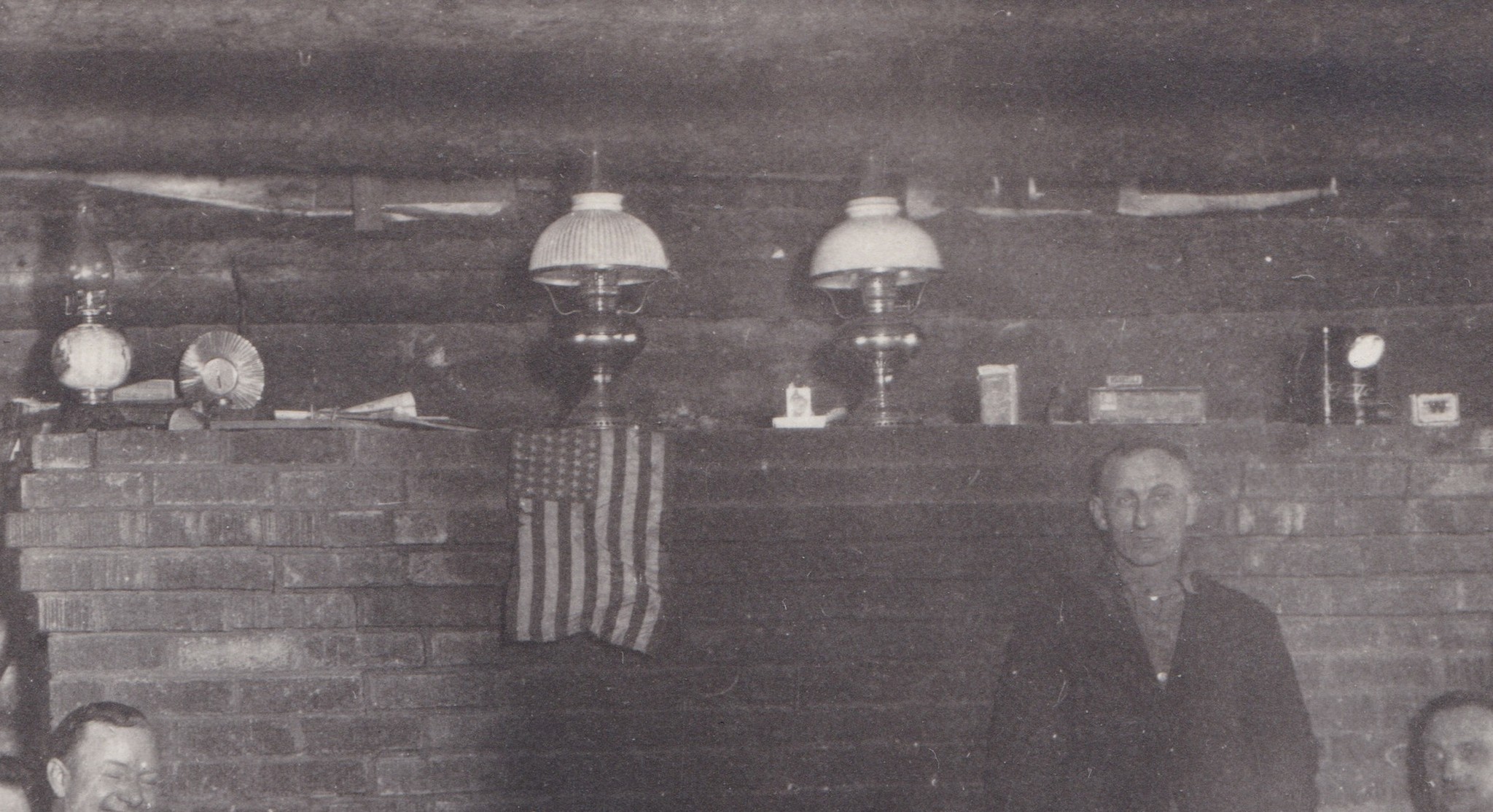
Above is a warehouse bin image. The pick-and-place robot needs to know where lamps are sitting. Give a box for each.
[809,139,947,430]
[49,199,137,430]
[527,139,673,427]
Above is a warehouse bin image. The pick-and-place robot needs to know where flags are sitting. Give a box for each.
[504,427,671,658]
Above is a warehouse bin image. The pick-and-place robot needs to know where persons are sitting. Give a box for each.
[986,439,1318,812]
[0,702,159,812]
[1407,689,1493,812]
